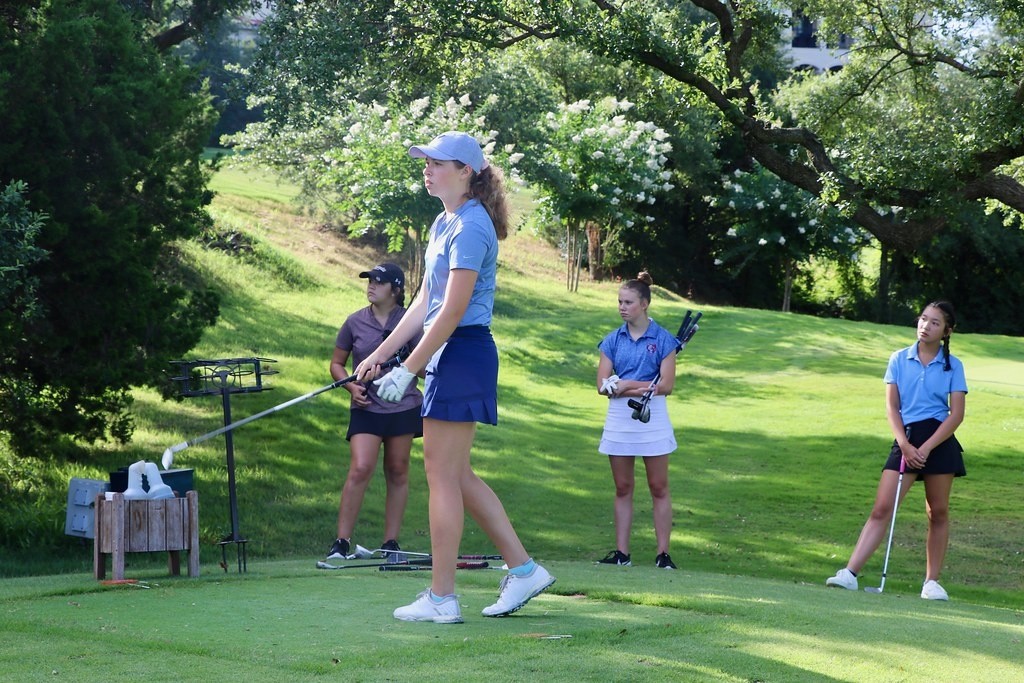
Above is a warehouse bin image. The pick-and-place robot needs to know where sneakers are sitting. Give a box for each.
[326,537,351,560]
[826,568,858,590]
[381,539,400,557]
[481,563,555,617]
[394,587,465,625]
[655,552,678,570]
[921,578,949,602]
[596,550,632,565]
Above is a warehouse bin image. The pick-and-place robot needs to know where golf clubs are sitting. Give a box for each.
[161,355,403,471]
[355,544,505,560]
[626,309,703,423]
[361,278,424,396]
[378,564,509,570]
[863,425,912,594]
[387,552,490,568]
[314,559,430,569]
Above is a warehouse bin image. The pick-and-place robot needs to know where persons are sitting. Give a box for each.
[326,264,423,561]
[826,302,967,600]
[353,130,557,624]
[593,270,678,569]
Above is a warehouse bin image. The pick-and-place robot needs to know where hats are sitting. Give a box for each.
[409,131,484,176]
[360,262,405,287]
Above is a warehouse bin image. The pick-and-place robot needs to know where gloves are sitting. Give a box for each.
[372,362,417,402]
[599,375,623,394]
